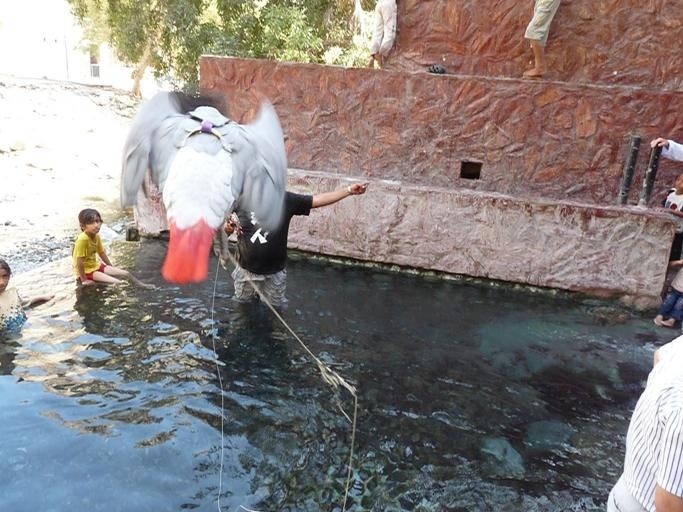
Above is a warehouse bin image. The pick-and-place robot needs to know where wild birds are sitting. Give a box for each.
[117,88,290,284]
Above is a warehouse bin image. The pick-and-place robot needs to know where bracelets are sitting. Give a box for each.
[348,186,352,195]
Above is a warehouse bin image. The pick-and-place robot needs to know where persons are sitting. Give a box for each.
[658,172,683,261]
[0,258,54,335]
[73,209,155,303]
[368,0,397,69]
[224,180,369,313]
[522,0,560,80]
[606,335,683,512]
[654,259,683,327]
[650,137,683,162]
